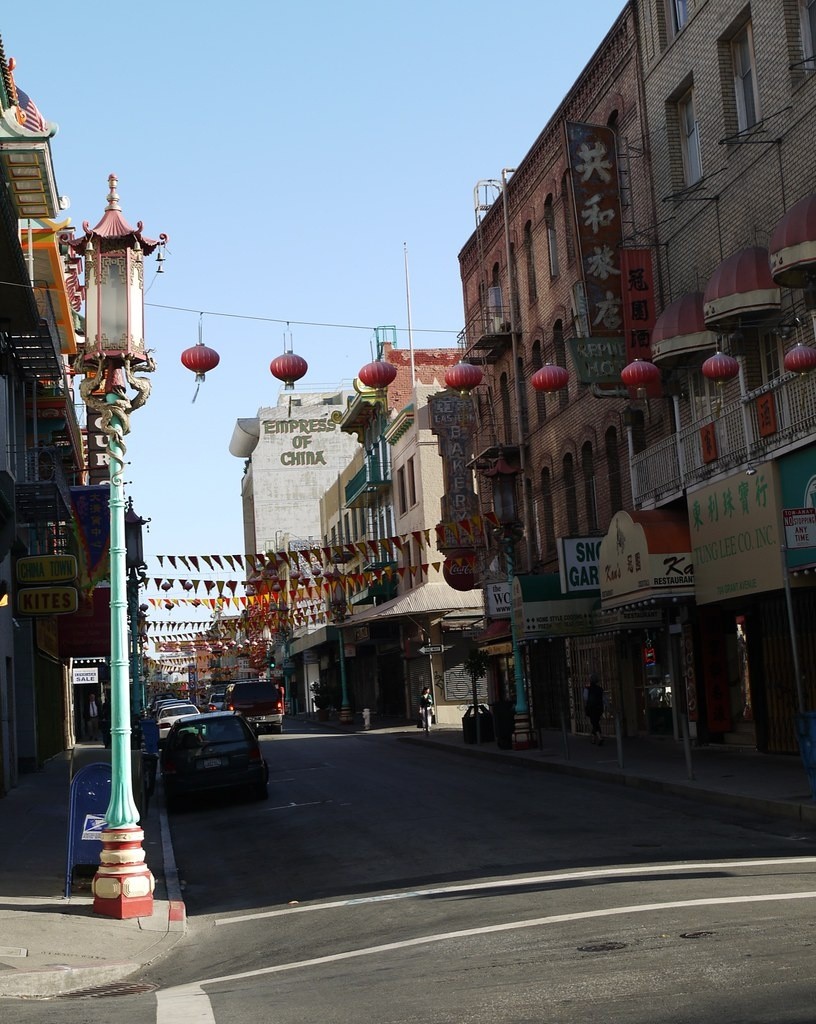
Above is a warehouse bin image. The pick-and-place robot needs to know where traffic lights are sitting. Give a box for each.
[270,658,275,668]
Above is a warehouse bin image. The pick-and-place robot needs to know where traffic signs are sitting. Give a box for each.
[417,645,443,654]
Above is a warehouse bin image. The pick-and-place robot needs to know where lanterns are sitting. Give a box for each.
[270,323,308,418]
[359,328,398,397]
[621,329,657,399]
[783,324,816,411]
[181,312,220,406]
[445,332,482,402]
[533,332,567,403]
[702,328,739,417]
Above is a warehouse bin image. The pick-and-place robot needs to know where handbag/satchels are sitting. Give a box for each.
[417,719,425,728]
[430,705,435,716]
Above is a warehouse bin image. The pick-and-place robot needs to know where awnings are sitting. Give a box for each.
[769,193,816,288]
[701,245,781,333]
[648,291,725,363]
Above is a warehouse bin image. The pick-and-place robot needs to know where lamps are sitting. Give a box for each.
[746,460,769,474]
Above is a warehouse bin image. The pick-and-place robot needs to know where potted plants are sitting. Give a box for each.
[308,683,332,721]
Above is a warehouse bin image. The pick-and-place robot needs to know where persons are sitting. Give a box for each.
[82,692,100,745]
[582,673,608,746]
[418,686,433,738]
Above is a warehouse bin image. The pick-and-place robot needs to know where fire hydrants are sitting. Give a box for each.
[362,708,371,730]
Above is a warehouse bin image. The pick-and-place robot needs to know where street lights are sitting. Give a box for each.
[481,452,537,751]
[123,494,153,748]
[327,562,354,725]
[61,172,168,920]
[278,602,293,714]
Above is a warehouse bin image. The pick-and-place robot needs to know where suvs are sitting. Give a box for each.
[221,677,283,734]
[207,692,225,712]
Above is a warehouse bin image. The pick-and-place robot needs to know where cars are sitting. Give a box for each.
[160,710,270,815]
[150,692,201,752]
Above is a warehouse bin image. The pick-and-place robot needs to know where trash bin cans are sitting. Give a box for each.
[463,705,495,744]
[142,753,159,797]
[69,747,146,875]
